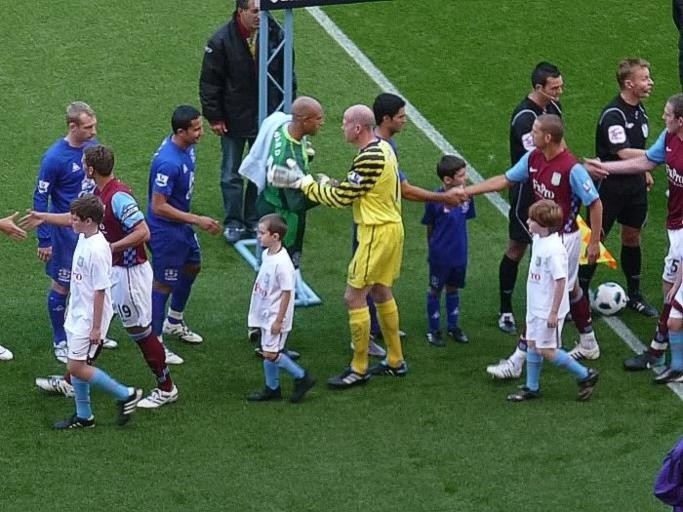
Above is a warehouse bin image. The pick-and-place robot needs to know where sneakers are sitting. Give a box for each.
[654,367,683,384]
[118,387,142,427]
[486,358,523,380]
[567,340,600,361]
[350,329,408,377]
[626,292,659,319]
[137,387,177,408]
[577,368,599,401]
[160,340,183,365]
[162,317,204,343]
[35,337,117,398]
[506,385,541,402]
[498,306,517,333]
[245,327,300,401]
[425,327,445,347]
[54,415,96,430]
[246,225,259,237]
[445,325,469,343]
[290,371,315,402]
[222,226,244,243]
[326,365,371,388]
[623,349,667,370]
[0,344,13,360]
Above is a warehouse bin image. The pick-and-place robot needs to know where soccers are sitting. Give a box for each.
[593,281,627,314]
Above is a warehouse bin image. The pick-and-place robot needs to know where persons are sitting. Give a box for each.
[19,144,180,409]
[200,2,297,244]
[52,193,143,430]
[247,94,476,386]
[33,101,118,364]
[0,212,27,362]
[245,213,316,402]
[464,56,683,402]
[143,105,223,365]
